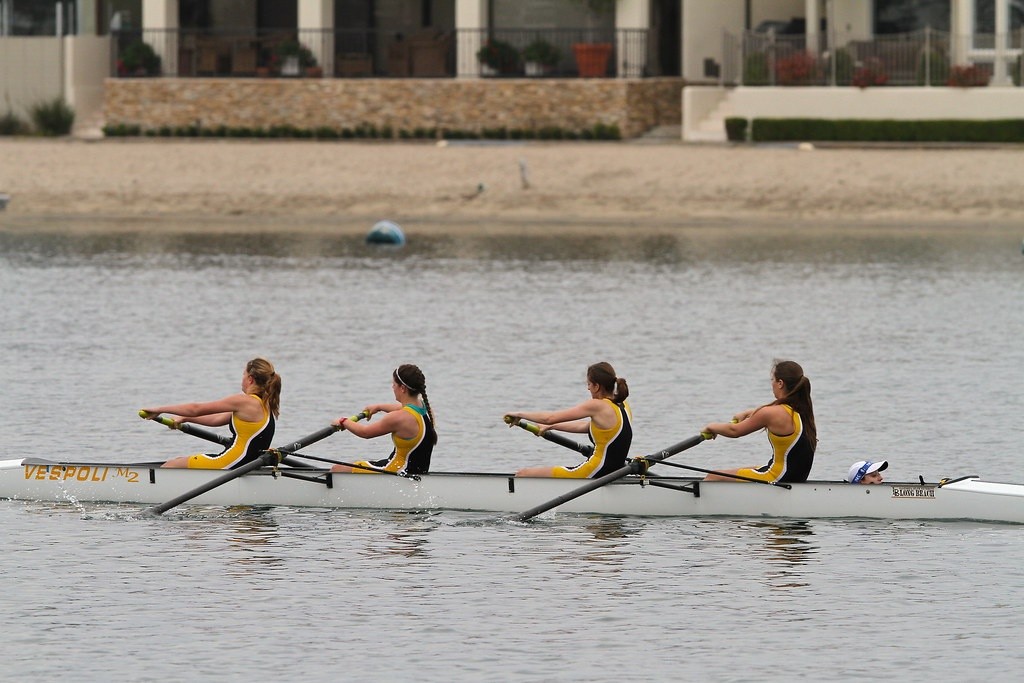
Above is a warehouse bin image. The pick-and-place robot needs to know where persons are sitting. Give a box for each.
[502,362,633,479]
[330,364,438,474]
[848,49,888,87]
[140,358,282,472]
[847,460,888,484]
[700,361,818,484]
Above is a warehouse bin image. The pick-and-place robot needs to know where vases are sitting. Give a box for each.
[574,42,612,77]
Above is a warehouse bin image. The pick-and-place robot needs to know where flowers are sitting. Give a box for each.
[778,50,823,86]
[948,64,990,87]
[852,72,890,88]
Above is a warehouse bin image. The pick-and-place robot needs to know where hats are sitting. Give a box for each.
[848,461,888,484]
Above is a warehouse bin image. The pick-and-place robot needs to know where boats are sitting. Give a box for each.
[0,459,1023,527]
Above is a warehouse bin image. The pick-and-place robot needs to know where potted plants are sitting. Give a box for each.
[123,41,154,77]
[522,38,562,74]
[277,39,303,76]
[476,37,520,76]
[824,48,853,85]
[914,45,948,84]
[254,45,271,76]
[303,55,323,77]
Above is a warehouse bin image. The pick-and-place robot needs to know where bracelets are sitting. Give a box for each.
[339,417,347,428]
